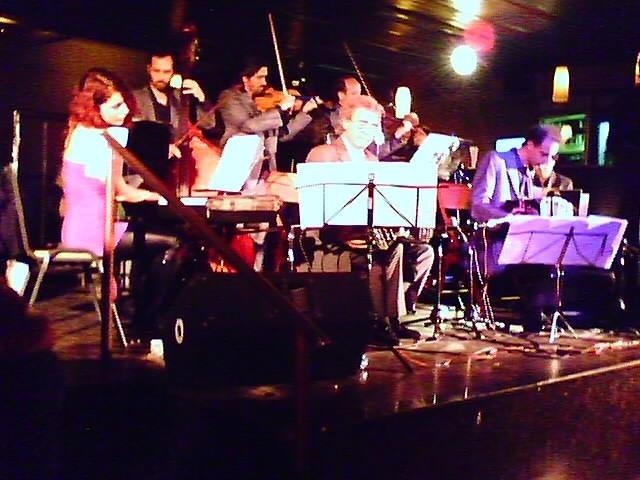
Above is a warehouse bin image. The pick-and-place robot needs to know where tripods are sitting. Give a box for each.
[359,184,578,371]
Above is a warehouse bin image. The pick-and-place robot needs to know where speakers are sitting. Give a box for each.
[166,272,373,384]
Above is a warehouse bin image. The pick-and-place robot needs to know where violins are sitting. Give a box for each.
[255,85,338,111]
[382,102,430,138]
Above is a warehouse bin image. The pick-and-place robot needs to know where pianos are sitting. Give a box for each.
[121,193,281,222]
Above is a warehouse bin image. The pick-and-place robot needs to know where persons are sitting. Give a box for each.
[306,78,469,340]
[218,59,318,192]
[472,123,572,339]
[70,50,215,339]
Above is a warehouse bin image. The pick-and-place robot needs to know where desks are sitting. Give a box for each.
[121,203,280,345]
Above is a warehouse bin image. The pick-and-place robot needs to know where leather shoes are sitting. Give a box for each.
[366,320,422,347]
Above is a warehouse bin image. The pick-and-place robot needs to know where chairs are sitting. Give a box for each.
[10,159,129,349]
[436,182,523,331]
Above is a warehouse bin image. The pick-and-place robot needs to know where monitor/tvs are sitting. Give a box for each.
[537,112,591,167]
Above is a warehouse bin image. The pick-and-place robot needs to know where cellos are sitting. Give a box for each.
[168,23,222,197]
